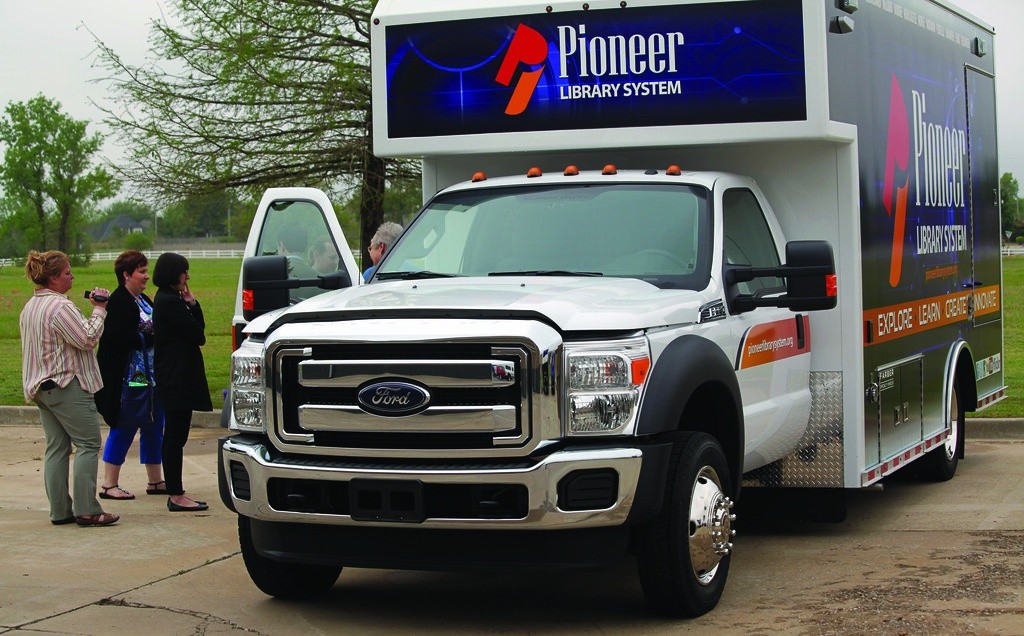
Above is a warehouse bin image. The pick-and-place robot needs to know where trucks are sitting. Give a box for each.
[217,0,1010,619]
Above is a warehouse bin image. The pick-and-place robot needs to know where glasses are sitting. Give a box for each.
[367,246,374,253]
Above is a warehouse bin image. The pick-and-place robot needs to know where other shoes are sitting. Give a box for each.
[167,497,209,512]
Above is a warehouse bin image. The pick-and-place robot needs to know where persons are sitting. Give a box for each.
[308,235,339,274]
[18,249,120,528]
[362,222,420,285]
[93,250,186,499]
[152,252,209,512]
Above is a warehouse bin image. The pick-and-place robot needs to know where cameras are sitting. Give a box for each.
[84,291,91,299]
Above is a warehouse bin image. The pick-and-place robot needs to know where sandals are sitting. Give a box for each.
[76,513,120,526]
[99,485,135,500]
[51,516,75,525]
[146,480,185,495]
